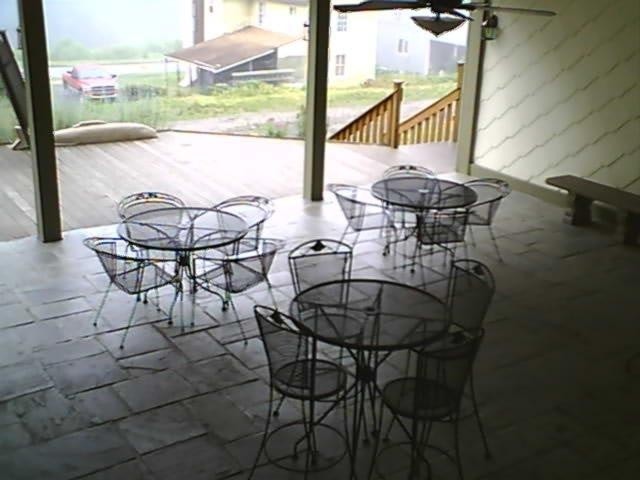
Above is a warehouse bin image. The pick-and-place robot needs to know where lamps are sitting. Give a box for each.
[408,7,467,36]
[477,10,500,40]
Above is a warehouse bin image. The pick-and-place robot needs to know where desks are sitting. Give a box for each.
[289,278,454,480]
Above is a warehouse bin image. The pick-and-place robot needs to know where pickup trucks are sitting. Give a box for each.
[61,62,118,102]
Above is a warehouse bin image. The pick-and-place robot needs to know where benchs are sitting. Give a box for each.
[537,170,640,238]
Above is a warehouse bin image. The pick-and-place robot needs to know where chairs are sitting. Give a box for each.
[382,258,498,444]
[274,237,354,416]
[327,164,513,298]
[247,305,358,479]
[365,327,493,480]
[82,190,285,351]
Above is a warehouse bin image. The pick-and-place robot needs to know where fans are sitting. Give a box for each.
[326,0,564,26]
